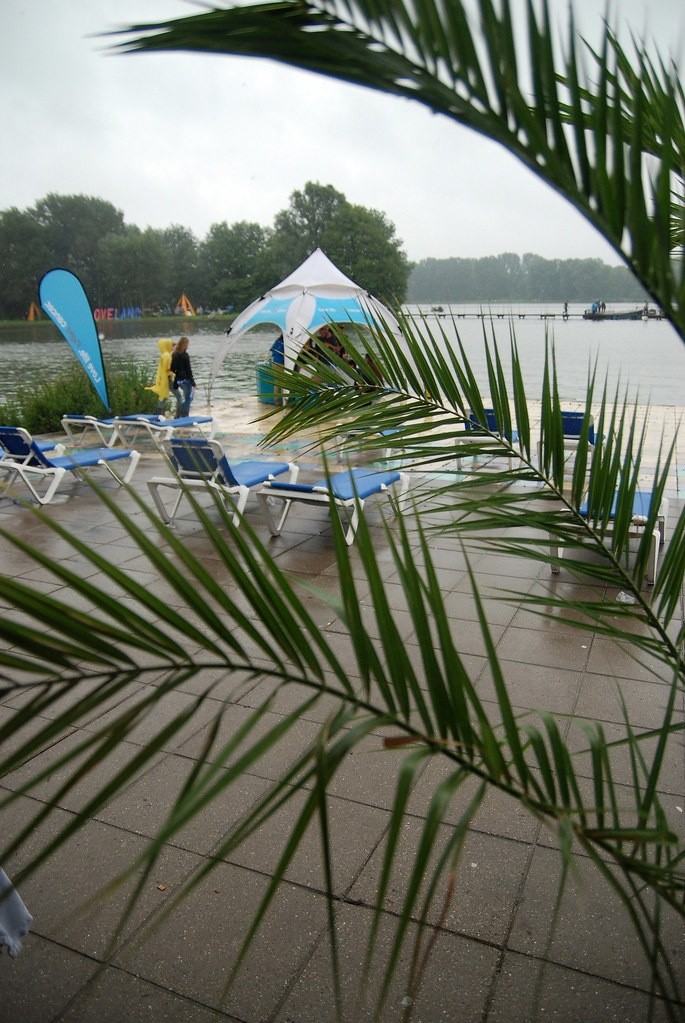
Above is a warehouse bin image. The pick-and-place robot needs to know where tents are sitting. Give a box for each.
[202,245,407,409]
[173,291,196,316]
[26,300,43,321]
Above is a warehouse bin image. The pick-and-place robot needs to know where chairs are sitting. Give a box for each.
[146,437,300,539]
[0,427,141,506]
[548,489,669,587]
[255,468,411,548]
[536,412,606,478]
[60,414,167,448]
[334,422,407,466]
[111,415,214,451]
[454,408,527,477]
[0,436,67,458]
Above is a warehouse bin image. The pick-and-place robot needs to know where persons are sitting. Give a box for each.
[643,299,649,315]
[591,298,606,315]
[154,338,183,422]
[562,300,569,314]
[268,322,384,410]
[168,336,200,418]
